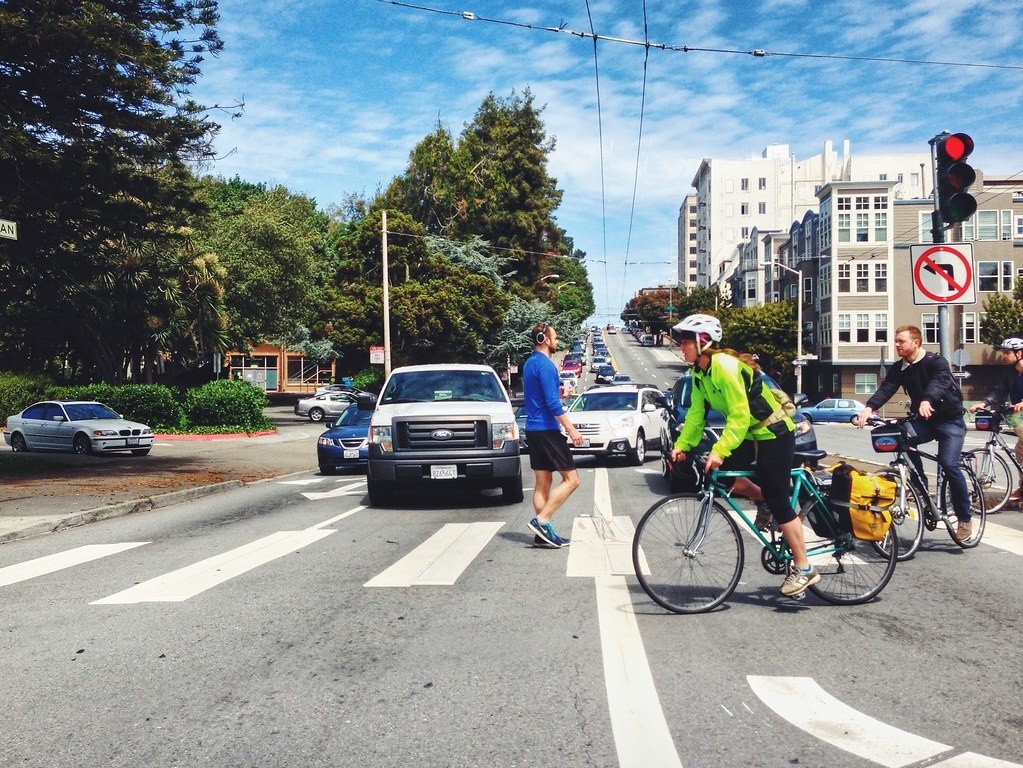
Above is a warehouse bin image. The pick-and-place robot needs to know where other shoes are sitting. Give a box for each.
[1009,488,1022,500]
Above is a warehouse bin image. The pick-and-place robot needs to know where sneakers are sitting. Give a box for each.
[897,488,930,501]
[534,534,570,547]
[753,499,772,530]
[527,517,561,548]
[779,564,822,596]
[955,519,972,542]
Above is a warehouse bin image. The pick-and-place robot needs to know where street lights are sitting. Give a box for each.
[761,260,803,409]
[558,281,577,294]
[668,279,690,319]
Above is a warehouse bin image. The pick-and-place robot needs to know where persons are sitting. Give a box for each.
[969,338,1023,500]
[667,314,822,597]
[859,326,973,542]
[522,323,584,550]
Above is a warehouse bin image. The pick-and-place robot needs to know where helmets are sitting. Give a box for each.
[999,338,1023,350]
[672,314,722,343]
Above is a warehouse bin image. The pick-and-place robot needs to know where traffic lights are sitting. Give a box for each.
[936,133,978,222]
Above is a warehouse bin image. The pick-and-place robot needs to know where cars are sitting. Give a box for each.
[317,402,376,476]
[558,319,674,409]
[797,398,881,427]
[2,399,155,456]
[513,406,529,450]
[294,390,359,422]
[560,382,668,467]
[313,384,378,402]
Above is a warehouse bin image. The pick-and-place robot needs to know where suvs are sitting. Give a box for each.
[655,368,818,496]
[356,364,526,507]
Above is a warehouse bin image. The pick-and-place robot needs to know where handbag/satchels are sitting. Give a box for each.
[792,461,898,542]
[669,454,703,492]
[975,410,1000,431]
[870,419,908,452]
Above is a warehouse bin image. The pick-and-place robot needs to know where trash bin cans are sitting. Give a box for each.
[342,377,351,386]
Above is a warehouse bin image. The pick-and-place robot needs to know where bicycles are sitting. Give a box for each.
[958,401,1023,515]
[868,411,987,561]
[632,448,901,615]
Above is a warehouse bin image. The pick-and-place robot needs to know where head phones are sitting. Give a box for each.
[536,324,546,344]
